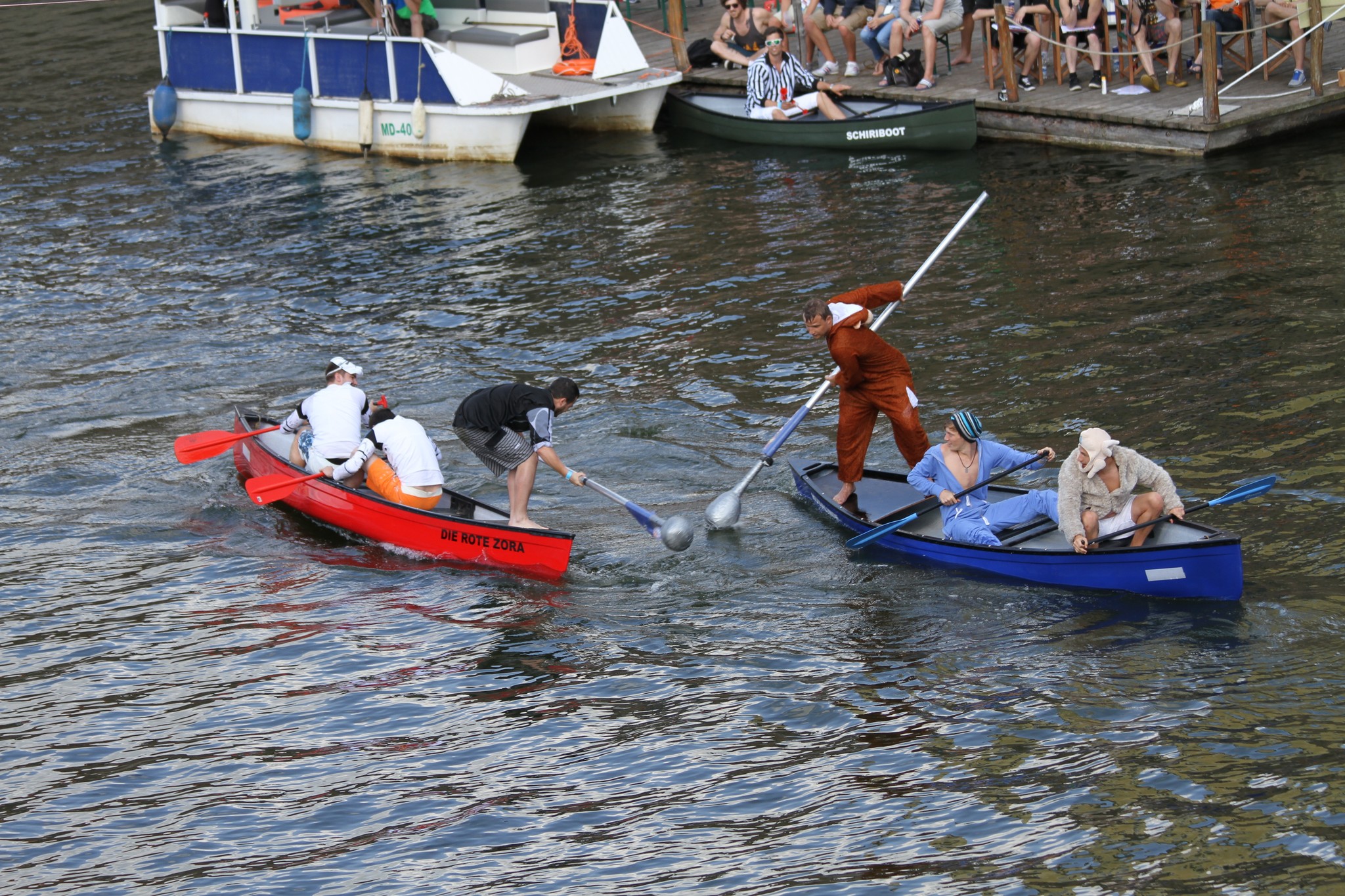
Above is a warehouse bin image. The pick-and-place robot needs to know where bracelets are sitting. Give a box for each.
[564,471,574,480]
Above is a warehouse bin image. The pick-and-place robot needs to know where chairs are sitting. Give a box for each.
[933,0,1312,90]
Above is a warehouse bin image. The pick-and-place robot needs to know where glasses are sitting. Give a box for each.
[725,3,738,9]
[765,39,782,47]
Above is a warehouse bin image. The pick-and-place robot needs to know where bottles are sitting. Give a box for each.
[1186,59,1193,76]
[867,17,876,30]
[1133,55,1138,72]
[1112,46,1118,53]
[1041,55,1047,79]
[1113,52,1120,72]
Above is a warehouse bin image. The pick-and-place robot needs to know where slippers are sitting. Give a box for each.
[1188,62,1202,73]
[914,79,937,90]
[1217,78,1225,84]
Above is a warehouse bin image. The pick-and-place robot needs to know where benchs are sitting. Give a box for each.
[991,512,1135,548]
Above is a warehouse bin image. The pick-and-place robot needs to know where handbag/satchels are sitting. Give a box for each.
[883,48,923,88]
[687,38,723,69]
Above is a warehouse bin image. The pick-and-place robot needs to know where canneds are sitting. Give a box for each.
[1133,58,1138,74]
[914,18,922,32]
[1186,59,1193,75]
[866,16,873,22]
[1112,47,1119,53]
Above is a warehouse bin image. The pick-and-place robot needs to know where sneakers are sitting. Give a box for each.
[1165,70,1187,87]
[724,60,744,70]
[1323,21,1332,32]
[1018,74,1035,91]
[1088,73,1101,88]
[1068,78,1082,91]
[844,62,860,77]
[1001,81,1006,93]
[1141,73,1160,93]
[1287,70,1306,87]
[812,61,839,77]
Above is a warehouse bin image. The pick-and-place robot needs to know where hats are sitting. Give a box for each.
[951,411,982,443]
[1077,427,1120,479]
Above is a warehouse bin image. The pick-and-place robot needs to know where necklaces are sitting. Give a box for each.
[957,441,978,472]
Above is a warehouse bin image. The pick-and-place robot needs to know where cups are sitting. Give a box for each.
[913,18,922,32]
[1008,1,1015,16]
[783,11,793,31]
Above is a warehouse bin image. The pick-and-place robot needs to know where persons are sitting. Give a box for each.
[1052,425,1183,554]
[280,356,443,510]
[802,282,938,510]
[370,0,1329,117]
[453,377,586,527]
[908,412,1060,548]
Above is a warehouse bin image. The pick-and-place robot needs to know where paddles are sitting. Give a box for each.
[174,419,280,466]
[847,448,1051,549]
[1080,475,1278,546]
[237,465,327,506]
